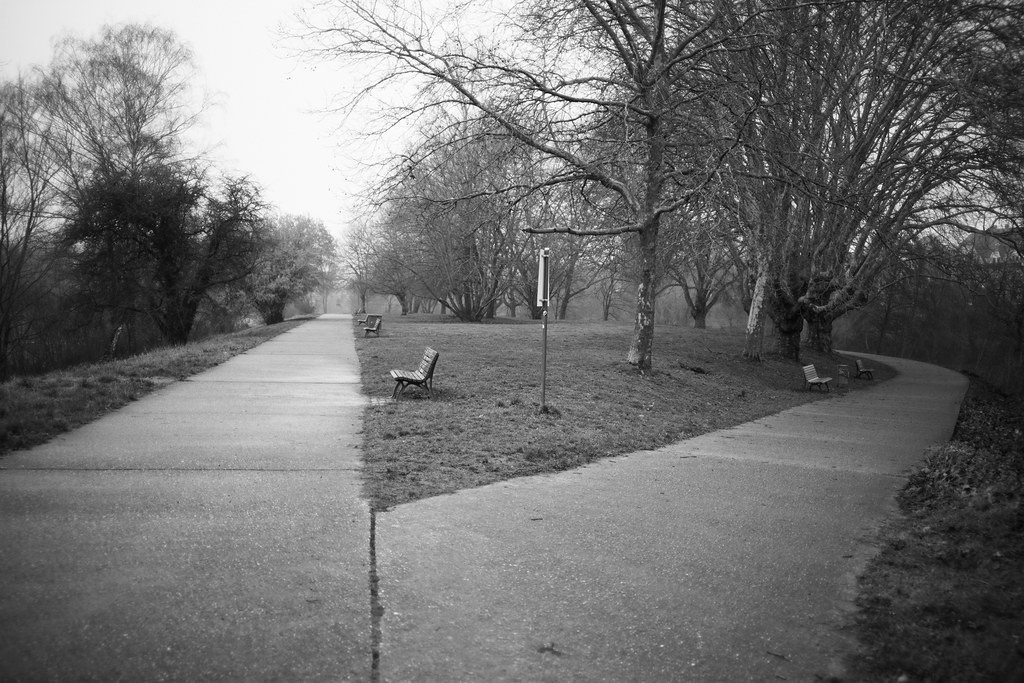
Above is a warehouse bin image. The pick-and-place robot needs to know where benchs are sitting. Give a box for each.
[363,317,381,338]
[389,347,439,402]
[357,316,368,326]
[802,363,832,394]
[854,360,875,380]
